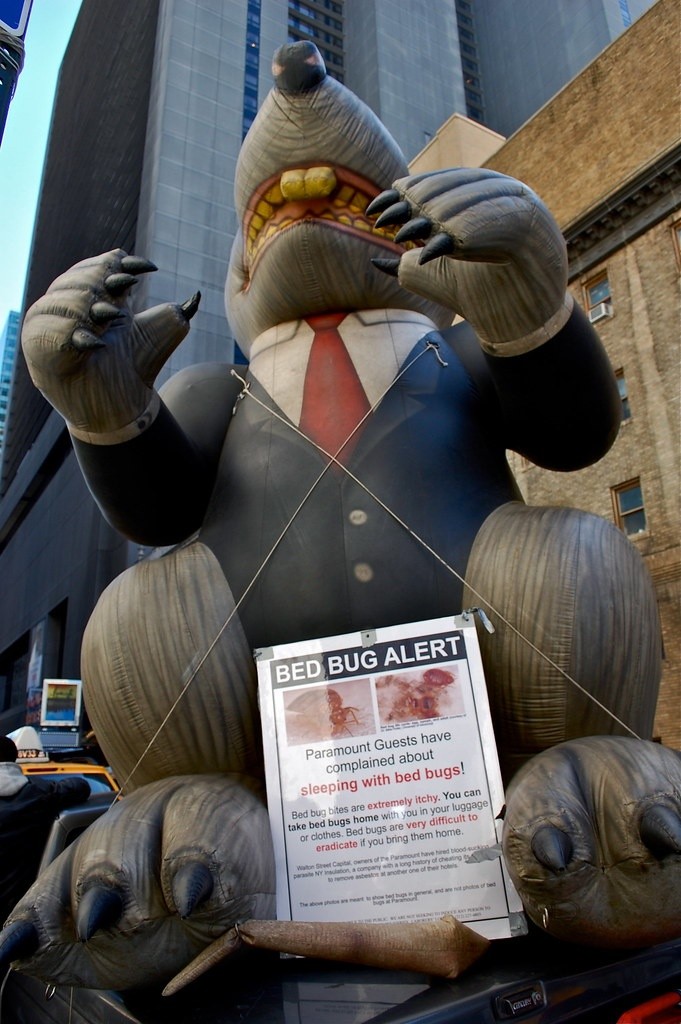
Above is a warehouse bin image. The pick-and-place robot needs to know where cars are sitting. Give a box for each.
[5,726,124,800]
[1,792,681,1024]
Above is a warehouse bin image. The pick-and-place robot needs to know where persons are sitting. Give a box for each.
[0,736,91,932]
